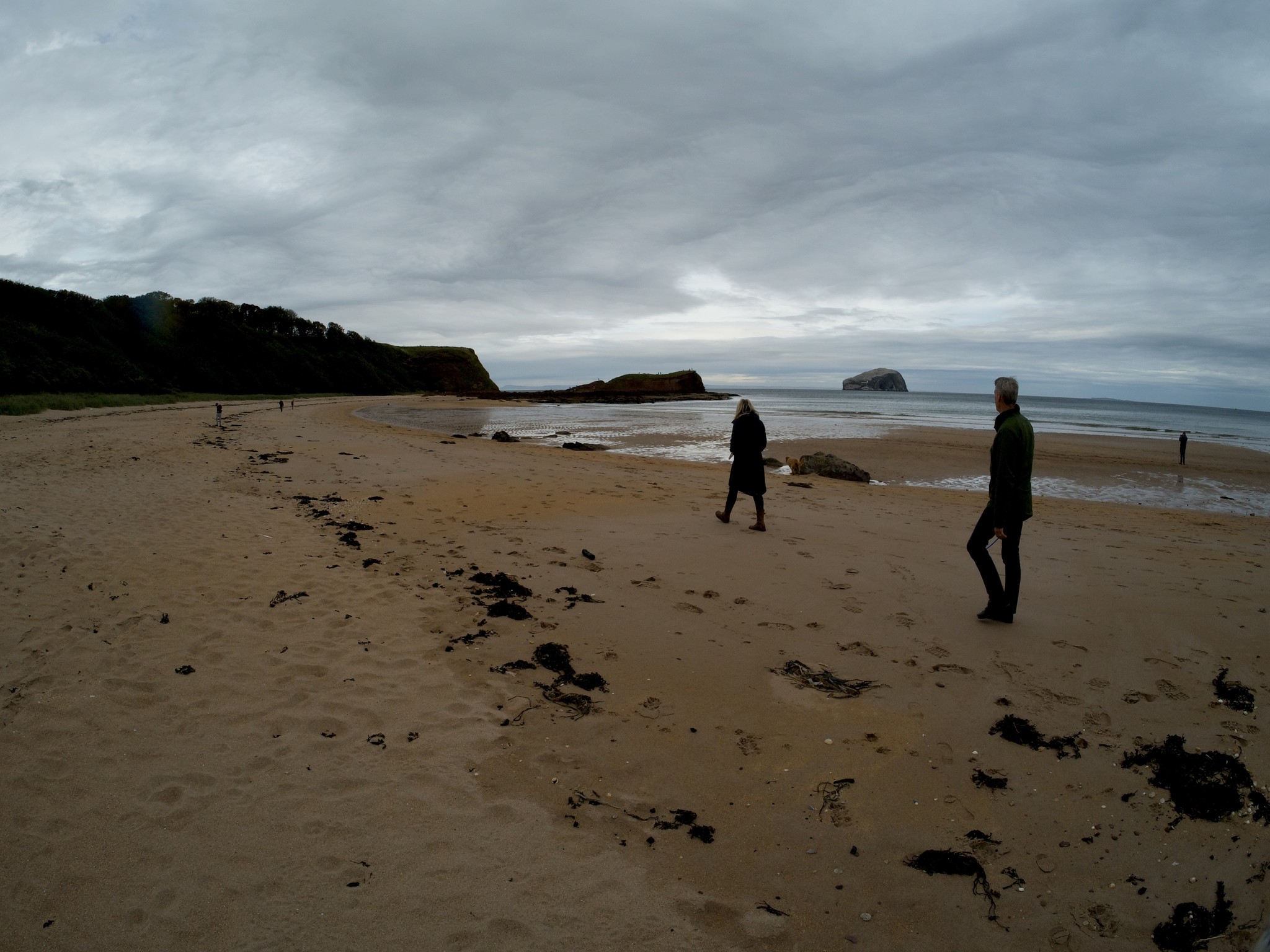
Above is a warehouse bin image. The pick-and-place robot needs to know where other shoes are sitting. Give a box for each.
[715,510,730,523]
[987,611,1014,624]
[749,521,766,532]
[977,597,1012,619]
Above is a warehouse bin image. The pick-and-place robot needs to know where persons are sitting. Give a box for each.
[216,403,222,427]
[1179,432,1187,465]
[715,399,767,532]
[966,377,1034,623]
[279,400,284,412]
[291,400,294,410]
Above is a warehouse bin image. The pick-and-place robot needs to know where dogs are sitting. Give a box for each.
[785,455,806,475]
